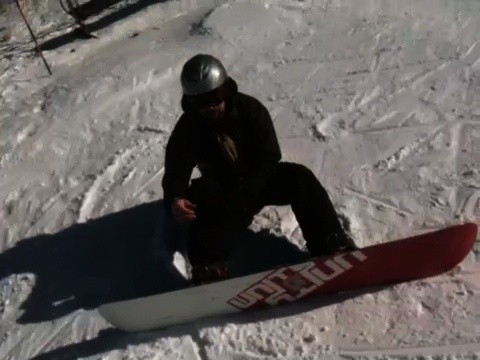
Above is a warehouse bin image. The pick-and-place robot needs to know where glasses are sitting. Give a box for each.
[183,87,227,107]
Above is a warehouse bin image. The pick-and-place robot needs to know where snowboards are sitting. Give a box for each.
[99,222,477,332]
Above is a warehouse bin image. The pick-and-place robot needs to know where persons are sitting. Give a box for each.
[162,54,353,275]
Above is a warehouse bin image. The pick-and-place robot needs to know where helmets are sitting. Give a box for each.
[180,54,229,96]
